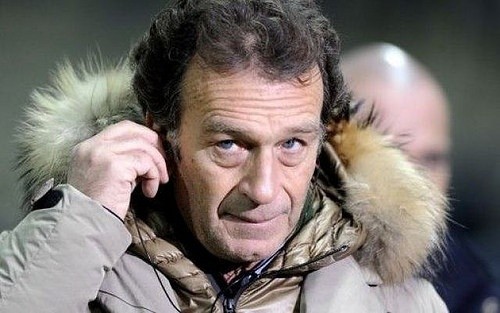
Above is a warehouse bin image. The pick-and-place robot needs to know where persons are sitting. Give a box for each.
[0,0,452,312]
[334,42,500,313]
